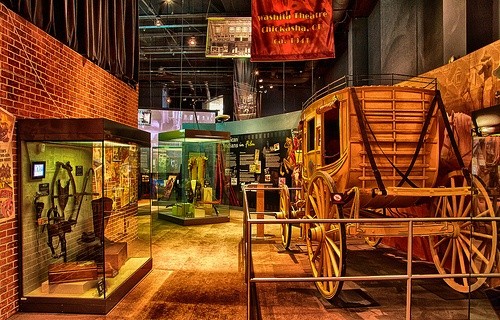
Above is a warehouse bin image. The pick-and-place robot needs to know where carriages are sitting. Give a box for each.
[276,73,500,301]
